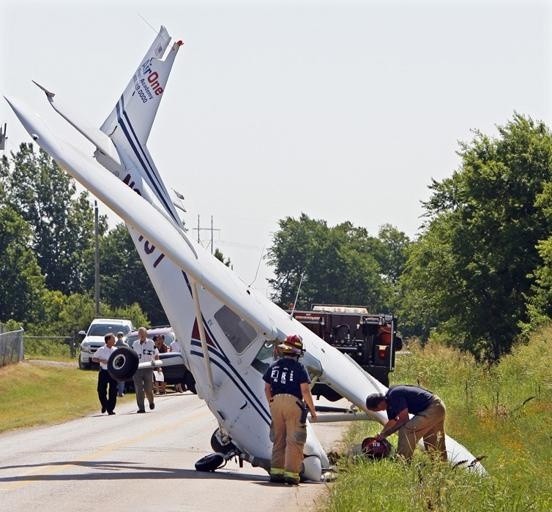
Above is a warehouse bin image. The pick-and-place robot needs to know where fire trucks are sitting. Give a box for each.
[280,303,402,402]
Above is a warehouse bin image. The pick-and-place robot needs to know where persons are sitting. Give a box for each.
[91,327,186,415]
[366,382,446,465]
[264,334,318,484]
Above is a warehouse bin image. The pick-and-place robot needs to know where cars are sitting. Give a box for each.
[79,318,189,394]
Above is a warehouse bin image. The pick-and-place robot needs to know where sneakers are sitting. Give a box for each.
[137,403,154,413]
[102,407,115,415]
[269,473,298,487]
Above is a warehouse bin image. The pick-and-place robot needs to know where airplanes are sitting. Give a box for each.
[2,24,492,482]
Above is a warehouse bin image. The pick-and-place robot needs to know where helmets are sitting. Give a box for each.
[361,437,391,461]
[283,333,304,349]
